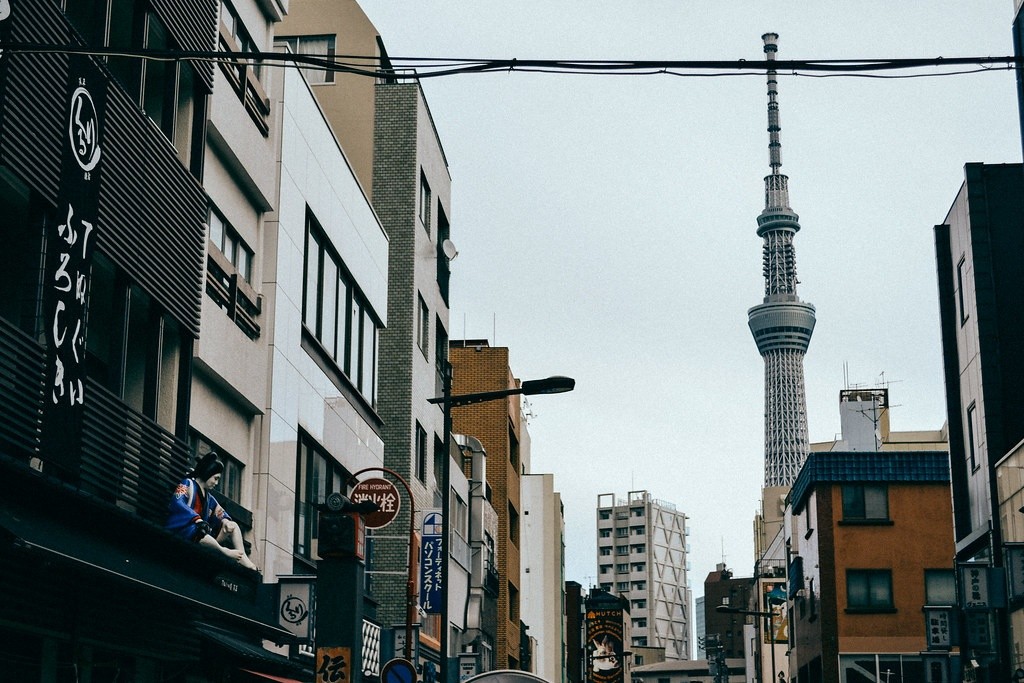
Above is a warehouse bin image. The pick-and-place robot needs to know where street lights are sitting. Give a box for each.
[587,651,633,683]
[440,376,576,683]
[716,605,776,683]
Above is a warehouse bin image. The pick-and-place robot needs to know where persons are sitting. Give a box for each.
[166,452,259,570]
